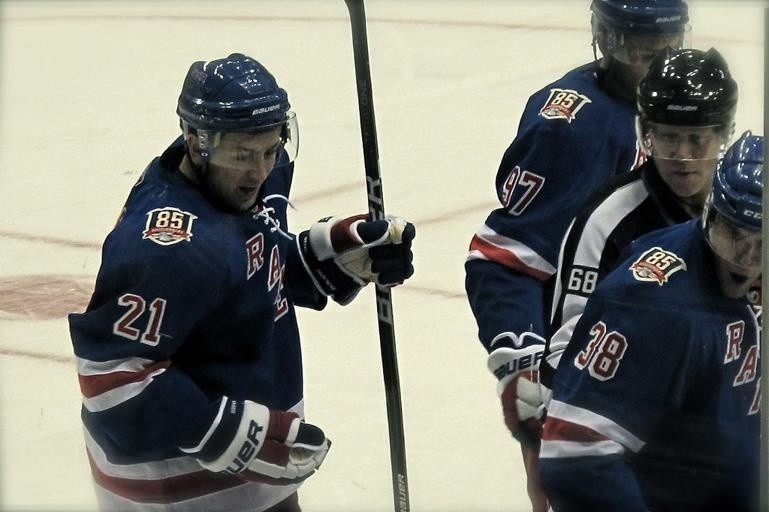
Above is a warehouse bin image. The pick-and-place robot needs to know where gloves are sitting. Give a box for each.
[486,344,547,451]
[296,213,415,306]
[180,395,331,485]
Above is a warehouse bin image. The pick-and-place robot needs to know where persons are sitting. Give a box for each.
[67,52,416,510]
[464,1,692,512]
[540,44,739,390]
[539,130,768,512]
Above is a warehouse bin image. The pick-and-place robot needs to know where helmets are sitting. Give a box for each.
[177,53,291,134]
[634,46,737,138]
[701,130,765,232]
[589,1,689,60]
